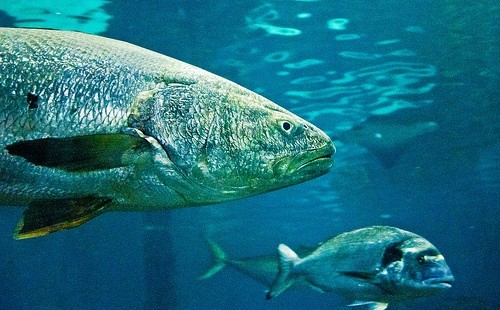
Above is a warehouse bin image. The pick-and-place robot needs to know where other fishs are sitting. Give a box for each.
[197,234,313,289]
[0,27,337,241]
[265,224,455,310]
